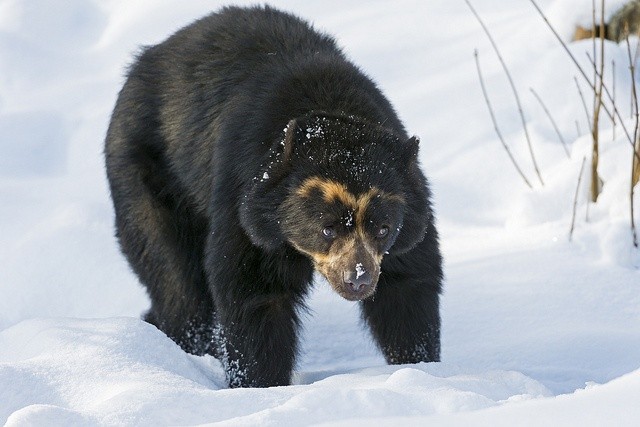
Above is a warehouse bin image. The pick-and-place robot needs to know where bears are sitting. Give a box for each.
[104,3,446,389]
